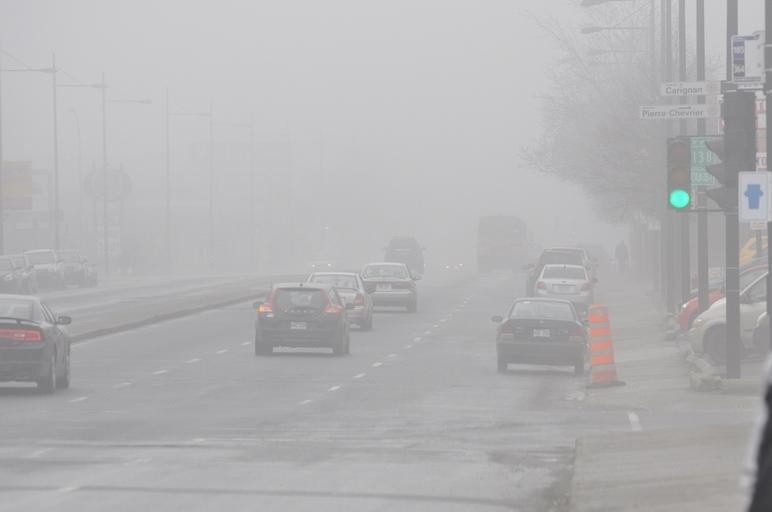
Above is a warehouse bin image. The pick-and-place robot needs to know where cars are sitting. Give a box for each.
[679,256,771,365]
[0,245,101,294]
[488,297,589,379]
[248,234,431,355]
[523,242,600,322]
[0,294,73,392]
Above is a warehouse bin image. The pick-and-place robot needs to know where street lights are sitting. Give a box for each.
[581,25,657,86]
[3,62,59,75]
[582,1,648,11]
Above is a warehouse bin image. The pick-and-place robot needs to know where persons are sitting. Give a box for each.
[615,239,631,276]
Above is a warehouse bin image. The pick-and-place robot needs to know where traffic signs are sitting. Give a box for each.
[639,103,720,119]
[661,78,721,95]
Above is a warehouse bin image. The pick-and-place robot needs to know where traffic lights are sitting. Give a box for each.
[665,132,693,213]
[704,135,728,216]
